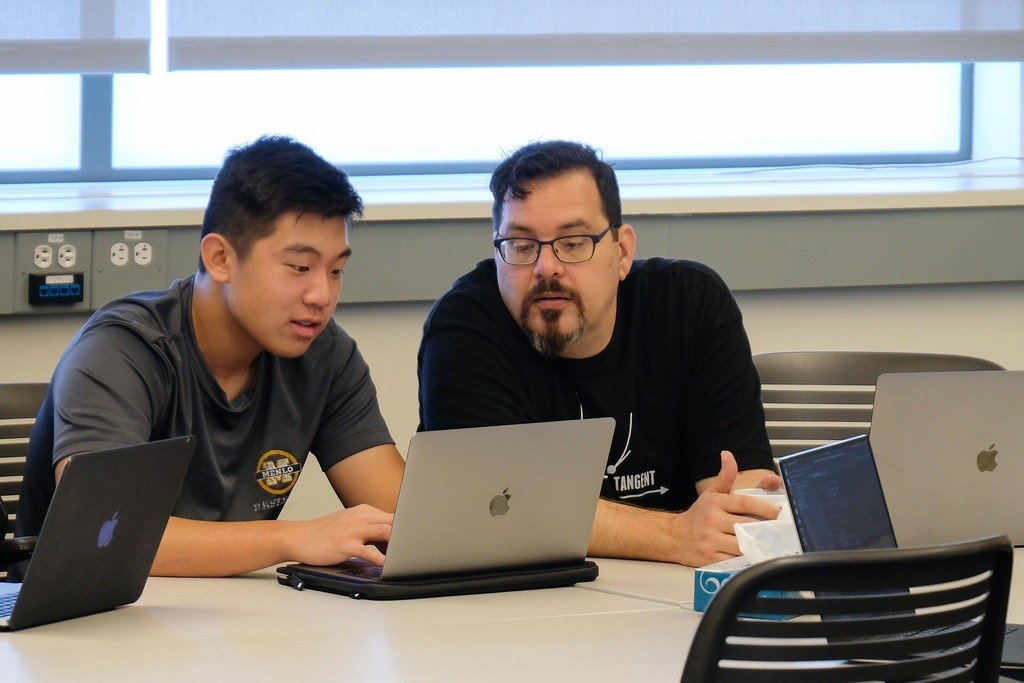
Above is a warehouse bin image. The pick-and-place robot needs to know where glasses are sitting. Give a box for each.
[493,222,612,266]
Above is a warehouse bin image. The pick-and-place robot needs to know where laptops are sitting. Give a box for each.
[867,369,1024,549]
[0,434,198,631]
[776,433,1024,674]
[287,416,617,584]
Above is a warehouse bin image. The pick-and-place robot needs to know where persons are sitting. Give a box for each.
[412,138,784,568]
[5,134,405,581]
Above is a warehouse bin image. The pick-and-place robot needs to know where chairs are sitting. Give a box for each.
[681,535,1015,683]
[0,382,51,579]
[751,351,1006,473]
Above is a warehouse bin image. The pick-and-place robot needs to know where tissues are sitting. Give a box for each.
[694,520,804,620]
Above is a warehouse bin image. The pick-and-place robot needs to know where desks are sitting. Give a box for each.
[0,544,1024,683]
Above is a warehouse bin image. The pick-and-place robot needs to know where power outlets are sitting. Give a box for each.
[14,231,91,313]
[91,229,169,314]
